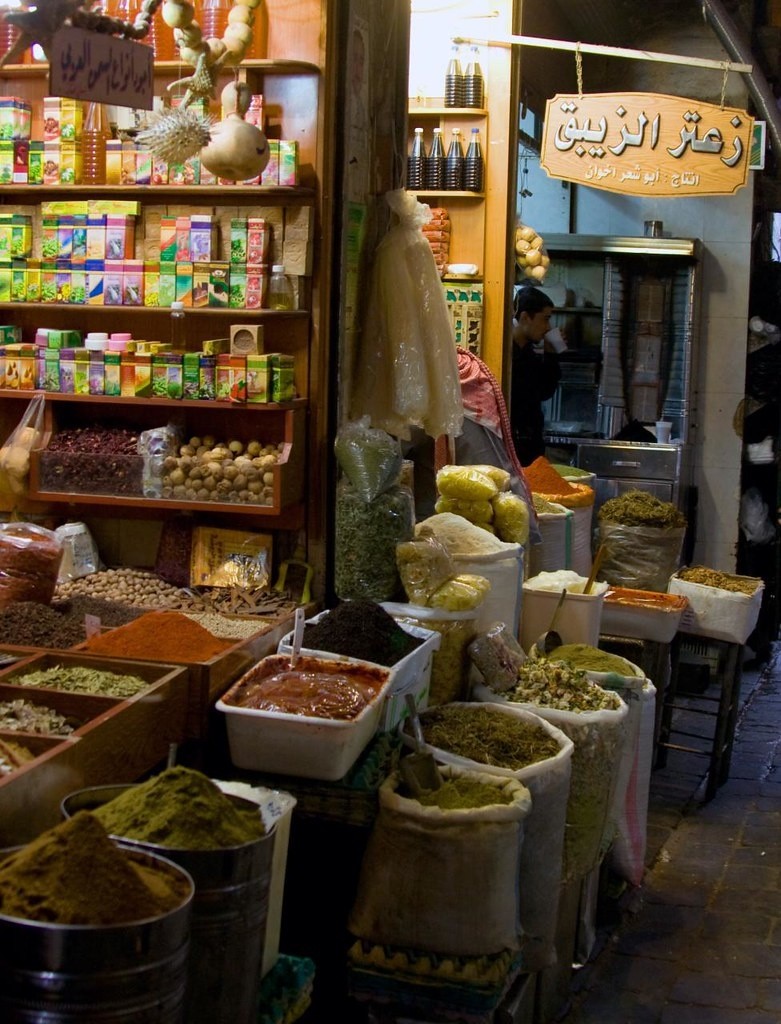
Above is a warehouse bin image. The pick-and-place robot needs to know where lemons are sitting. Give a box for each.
[516,226,550,283]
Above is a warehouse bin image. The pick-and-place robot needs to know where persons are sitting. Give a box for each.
[510,285,567,468]
[400,347,543,548]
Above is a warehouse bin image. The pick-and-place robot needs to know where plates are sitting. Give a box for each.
[649,442,676,448]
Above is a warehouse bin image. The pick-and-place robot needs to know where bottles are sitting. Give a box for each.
[79,101,106,184]
[444,45,462,108]
[167,302,188,356]
[0,0,269,62]
[463,46,483,108]
[444,128,465,191]
[428,129,446,191]
[408,128,428,191]
[269,265,291,311]
[465,127,484,191]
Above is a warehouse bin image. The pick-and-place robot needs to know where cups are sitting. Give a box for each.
[644,220,663,238]
[656,421,672,444]
[546,327,566,354]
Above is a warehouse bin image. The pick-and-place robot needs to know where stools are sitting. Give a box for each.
[597,633,658,682]
[651,629,746,805]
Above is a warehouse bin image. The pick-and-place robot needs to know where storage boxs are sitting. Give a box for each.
[0,646,190,789]
[441,283,484,359]
[0,728,84,854]
[600,585,689,645]
[214,653,398,783]
[206,777,298,981]
[667,565,765,646]
[523,575,610,657]
[277,609,442,734]
[247,732,402,833]
[0,590,324,743]
[0,96,296,404]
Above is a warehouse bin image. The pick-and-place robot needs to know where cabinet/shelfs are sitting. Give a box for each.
[0,58,326,533]
[540,445,696,480]
[515,232,704,444]
[407,0,525,436]
[592,477,698,569]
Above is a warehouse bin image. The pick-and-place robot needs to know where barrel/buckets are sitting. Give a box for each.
[595,394,626,441]
[0,842,197,1024]
[58,769,274,1024]
